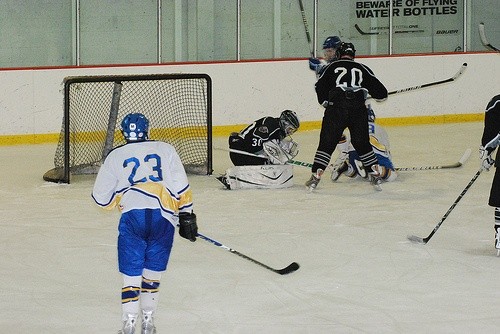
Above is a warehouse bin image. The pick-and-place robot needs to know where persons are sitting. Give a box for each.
[305,42,387,192]
[92,113,198,334]
[329,108,396,183]
[309,36,349,154]
[482,94,500,256]
[216,110,300,190]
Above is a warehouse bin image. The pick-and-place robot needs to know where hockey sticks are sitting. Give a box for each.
[177,223,300,275]
[479,22,500,53]
[355,23,425,35]
[299,0,320,80]
[407,167,483,244]
[394,148,472,171]
[213,146,348,170]
[366,63,468,100]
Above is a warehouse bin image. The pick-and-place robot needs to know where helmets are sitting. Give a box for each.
[367,108,375,122]
[336,42,356,58]
[322,36,342,50]
[279,110,299,135]
[120,113,150,140]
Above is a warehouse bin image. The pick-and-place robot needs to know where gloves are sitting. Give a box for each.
[479,146,495,171]
[309,58,326,74]
[178,209,198,242]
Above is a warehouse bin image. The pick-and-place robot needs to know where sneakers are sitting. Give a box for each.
[216,176,230,189]
[305,168,324,192]
[367,164,383,191]
[331,151,350,180]
[495,228,500,256]
[141,310,156,334]
[115,314,139,334]
[355,160,368,178]
